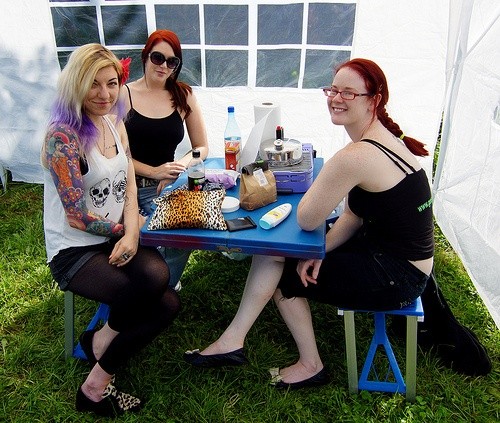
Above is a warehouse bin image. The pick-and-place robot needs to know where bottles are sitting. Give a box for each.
[259,203,292,230]
[188,149,206,191]
[224,106,242,171]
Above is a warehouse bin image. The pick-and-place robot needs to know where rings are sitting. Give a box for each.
[122,254,130,260]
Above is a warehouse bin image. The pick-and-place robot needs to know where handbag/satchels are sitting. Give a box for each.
[145,186,228,232]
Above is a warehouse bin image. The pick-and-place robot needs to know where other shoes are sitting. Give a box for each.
[183,348,249,366]
[74,382,145,415]
[79,329,100,366]
[269,365,332,388]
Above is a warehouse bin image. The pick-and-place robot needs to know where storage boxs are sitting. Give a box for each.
[225,141,242,171]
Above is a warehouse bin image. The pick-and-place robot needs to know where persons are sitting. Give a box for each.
[41,43,182,415]
[182,58,434,390]
[110,28,208,290]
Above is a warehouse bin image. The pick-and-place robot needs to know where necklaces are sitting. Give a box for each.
[97,122,105,156]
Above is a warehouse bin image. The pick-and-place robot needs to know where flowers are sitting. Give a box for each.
[119,56,132,87]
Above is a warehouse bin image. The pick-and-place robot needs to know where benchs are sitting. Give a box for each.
[337,295,426,405]
[63,290,109,361]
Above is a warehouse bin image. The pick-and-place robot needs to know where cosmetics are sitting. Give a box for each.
[221,196,240,212]
[260,202,292,229]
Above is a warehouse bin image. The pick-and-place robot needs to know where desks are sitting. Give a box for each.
[137,157,329,259]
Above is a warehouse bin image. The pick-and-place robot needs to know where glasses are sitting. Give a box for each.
[323,88,371,100]
[148,51,181,69]
